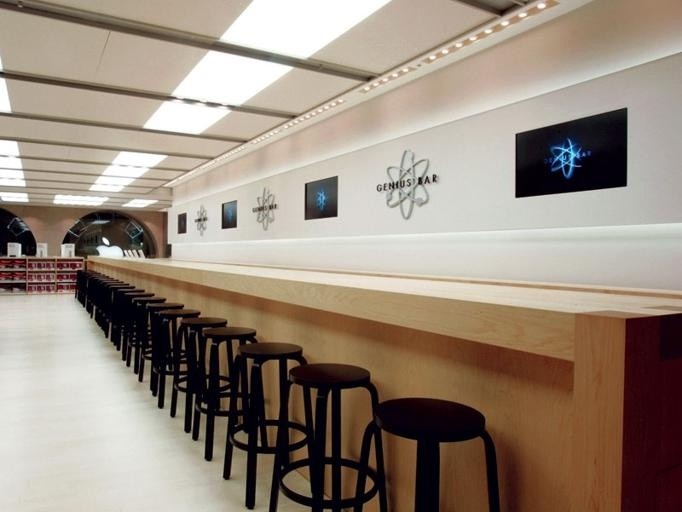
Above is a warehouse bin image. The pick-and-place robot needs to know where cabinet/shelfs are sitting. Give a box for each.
[26,258,57,294]
[56,257,83,295]
[1,259,26,292]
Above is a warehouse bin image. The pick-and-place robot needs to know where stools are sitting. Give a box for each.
[270,361,388,511]
[354,393,499,512]
